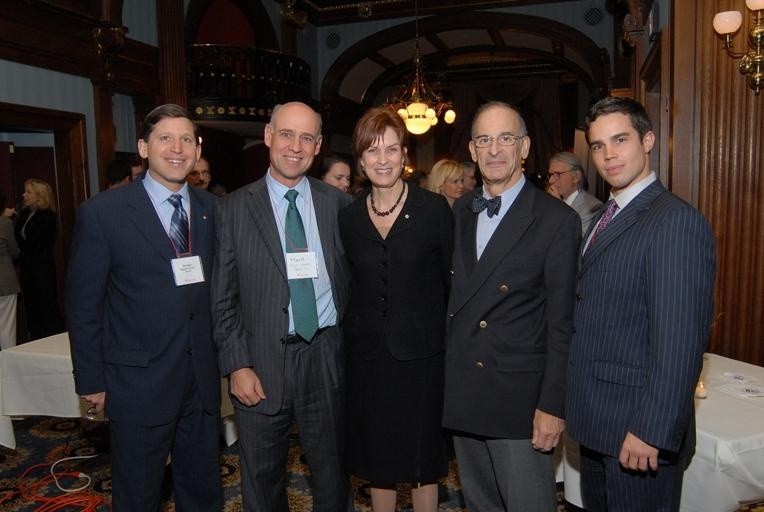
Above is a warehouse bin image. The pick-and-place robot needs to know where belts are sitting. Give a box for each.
[278,324,338,347]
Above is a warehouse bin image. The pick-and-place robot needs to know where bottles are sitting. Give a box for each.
[695,381,707,400]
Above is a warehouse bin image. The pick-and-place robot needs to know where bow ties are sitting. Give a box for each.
[471,192,502,219]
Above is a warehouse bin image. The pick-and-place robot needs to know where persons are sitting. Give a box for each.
[210,101,358,511]
[15,179,60,341]
[340,108,456,511]
[0,189,21,352]
[185,158,209,191]
[549,152,605,239]
[65,102,225,512]
[564,94,716,510]
[315,158,351,191]
[442,101,582,512]
[409,159,476,206]
[107,152,146,191]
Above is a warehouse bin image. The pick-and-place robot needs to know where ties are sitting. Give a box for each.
[582,197,619,259]
[280,190,321,345]
[164,193,190,254]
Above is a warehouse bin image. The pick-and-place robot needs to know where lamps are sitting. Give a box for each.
[711,0,764,97]
[377,1,457,138]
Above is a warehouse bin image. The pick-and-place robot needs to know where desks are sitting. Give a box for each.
[0,328,239,456]
[553,348,764,512]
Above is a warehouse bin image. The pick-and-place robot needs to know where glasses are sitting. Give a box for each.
[470,133,526,149]
[546,169,574,179]
